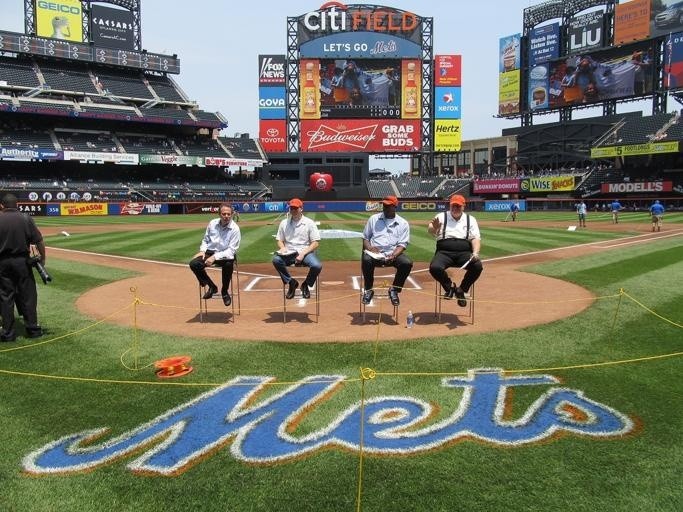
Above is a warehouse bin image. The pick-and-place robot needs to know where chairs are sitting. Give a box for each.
[0,66,267,191]
[366,179,471,199]
[577,113,683,189]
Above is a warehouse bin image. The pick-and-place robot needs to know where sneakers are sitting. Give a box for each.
[361,290,374,304]
[299,282,310,299]
[285,279,298,300]
[442,281,455,300]
[25,323,43,339]
[0,325,15,343]
[453,286,466,307]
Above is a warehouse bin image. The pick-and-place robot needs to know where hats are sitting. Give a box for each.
[378,195,398,207]
[448,194,465,206]
[288,198,303,208]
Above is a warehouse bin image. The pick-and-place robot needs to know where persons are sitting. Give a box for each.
[548,47,654,109]
[319,60,401,105]
[428,194,484,308]
[391,164,683,233]
[271,199,323,300]
[188,203,242,306]
[360,195,414,306]
[232,210,240,222]
[0,68,260,201]
[0,193,46,343]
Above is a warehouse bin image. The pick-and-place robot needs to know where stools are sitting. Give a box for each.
[359,265,398,325]
[435,267,474,324]
[283,263,319,324]
[198,259,240,324]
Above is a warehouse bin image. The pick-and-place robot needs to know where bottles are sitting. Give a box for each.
[301,71,316,115]
[406,311,413,329]
[404,64,416,114]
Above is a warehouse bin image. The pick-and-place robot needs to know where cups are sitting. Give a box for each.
[503,55,515,71]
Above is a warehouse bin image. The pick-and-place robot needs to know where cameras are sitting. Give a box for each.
[29,254,52,285]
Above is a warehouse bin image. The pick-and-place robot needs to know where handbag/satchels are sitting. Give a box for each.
[22,212,42,265]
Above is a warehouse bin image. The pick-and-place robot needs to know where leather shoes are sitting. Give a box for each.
[202,286,218,300]
[220,289,231,307]
[387,287,399,306]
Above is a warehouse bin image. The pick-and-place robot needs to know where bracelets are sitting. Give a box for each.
[393,255,397,261]
